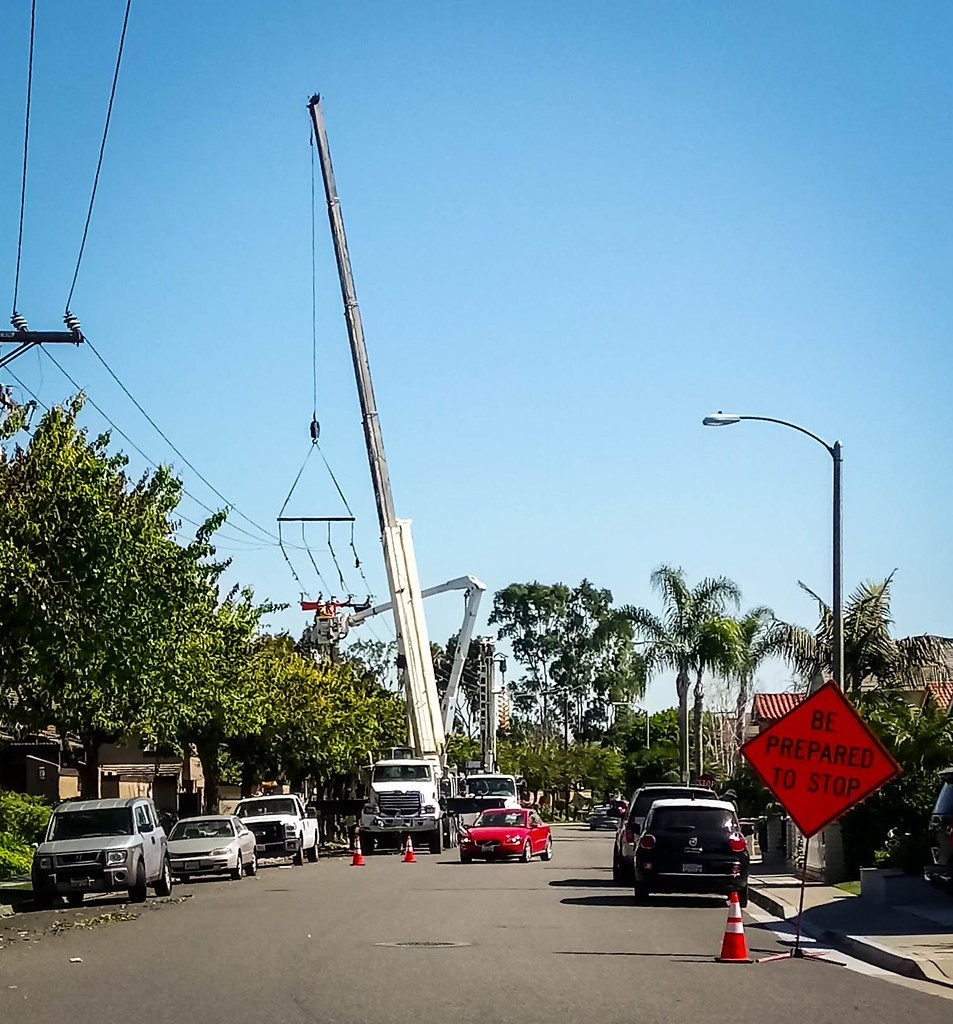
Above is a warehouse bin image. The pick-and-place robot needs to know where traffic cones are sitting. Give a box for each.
[350,833,365,866]
[714,892,754,962]
[401,835,417,863]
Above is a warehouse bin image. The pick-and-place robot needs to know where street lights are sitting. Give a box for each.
[539,689,576,818]
[611,701,651,749]
[702,409,846,694]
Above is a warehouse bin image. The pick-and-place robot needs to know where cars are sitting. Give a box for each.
[166,813,258,880]
[630,795,751,907]
[589,807,622,833]
[461,808,553,864]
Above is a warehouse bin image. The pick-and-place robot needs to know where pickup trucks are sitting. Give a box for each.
[233,795,320,865]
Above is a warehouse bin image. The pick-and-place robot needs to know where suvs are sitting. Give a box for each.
[32,796,175,902]
[922,766,952,899]
[612,782,720,890]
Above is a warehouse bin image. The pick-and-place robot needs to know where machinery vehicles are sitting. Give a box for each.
[283,91,453,855]
[313,576,523,832]
